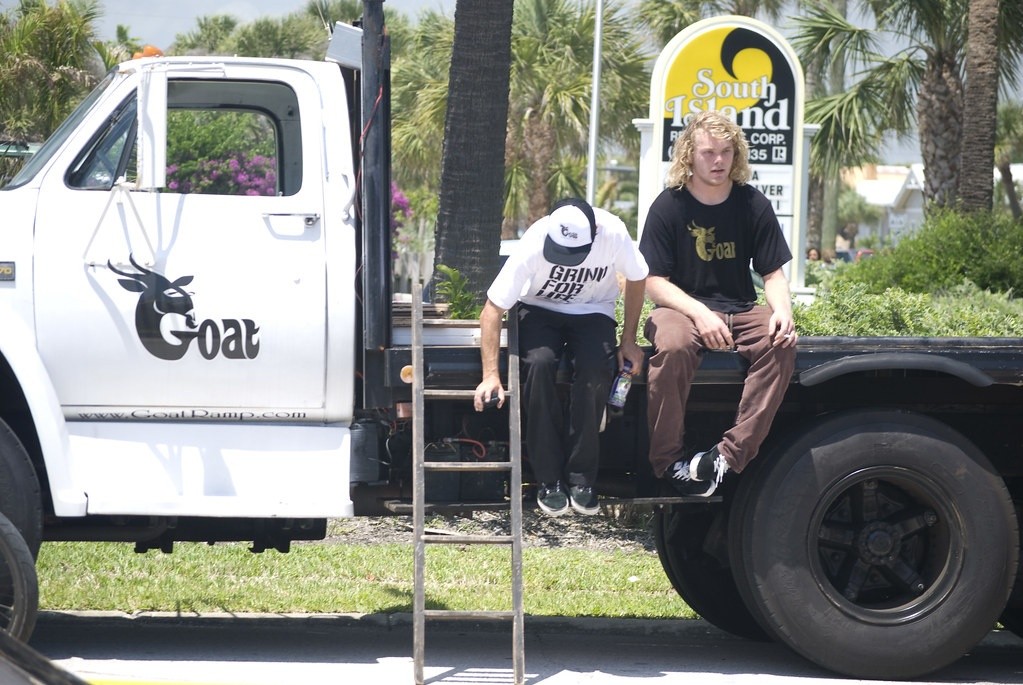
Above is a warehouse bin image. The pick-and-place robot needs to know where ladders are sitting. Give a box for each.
[411,287,524,685]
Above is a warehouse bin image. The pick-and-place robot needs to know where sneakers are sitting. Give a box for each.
[568,483,600,515]
[537,479,569,516]
[689,443,731,483]
[666,458,716,498]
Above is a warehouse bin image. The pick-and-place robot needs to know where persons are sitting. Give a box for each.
[474,196,650,517]
[640,111,801,497]
[835,228,852,264]
[806,248,828,268]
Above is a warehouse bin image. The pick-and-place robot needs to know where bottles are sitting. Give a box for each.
[607,361,632,410]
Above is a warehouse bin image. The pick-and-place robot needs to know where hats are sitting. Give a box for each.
[543,198,596,267]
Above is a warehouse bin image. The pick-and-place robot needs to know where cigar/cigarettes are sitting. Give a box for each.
[776,331,791,338]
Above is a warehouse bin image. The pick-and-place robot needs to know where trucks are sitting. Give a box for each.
[1,1,1023,685]
